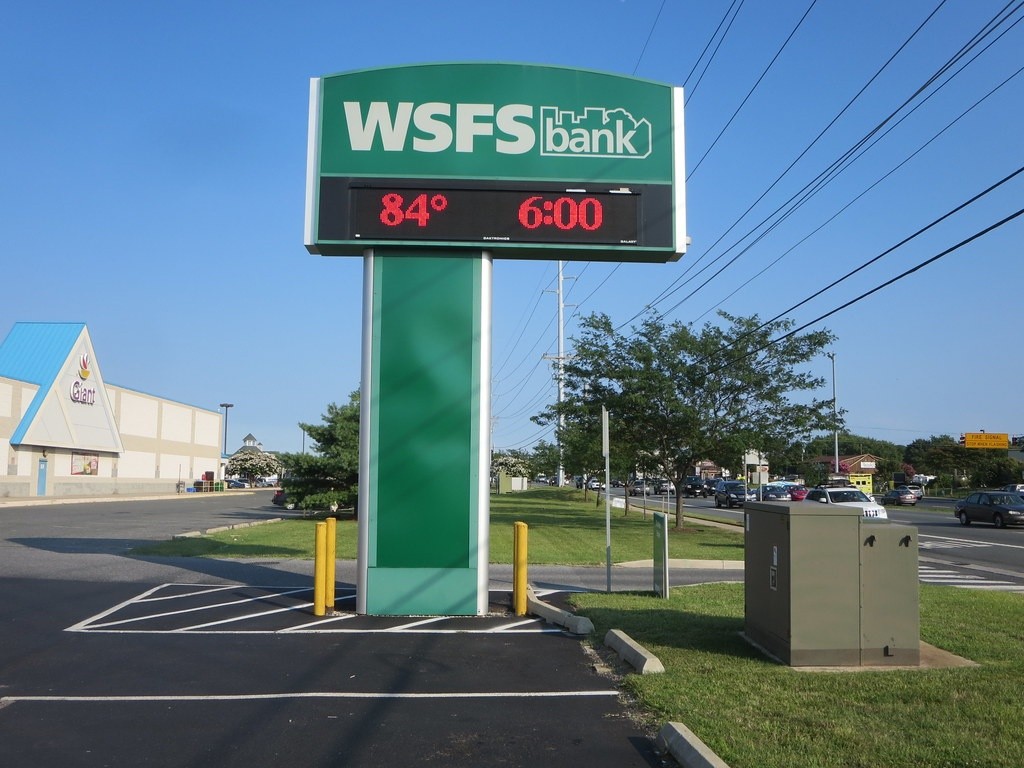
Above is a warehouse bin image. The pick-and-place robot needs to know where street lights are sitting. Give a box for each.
[220,403,234,454]
[827,352,838,473]
[980,430,986,458]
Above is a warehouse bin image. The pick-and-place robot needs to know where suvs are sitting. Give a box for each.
[684,475,708,498]
[714,481,757,509]
[576,478,583,488]
[612,478,628,487]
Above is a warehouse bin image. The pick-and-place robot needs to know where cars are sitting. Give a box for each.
[756,485,792,500]
[224,479,251,489]
[815,479,855,488]
[272,479,354,511]
[784,485,809,501]
[802,488,888,520]
[1000,484,1024,501]
[881,490,917,506]
[654,480,675,495]
[549,476,558,486]
[628,480,650,496]
[954,492,1024,529]
[588,479,605,491]
[536,476,546,482]
[704,479,725,496]
[896,485,923,500]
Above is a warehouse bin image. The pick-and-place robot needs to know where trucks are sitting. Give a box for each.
[256,478,277,486]
